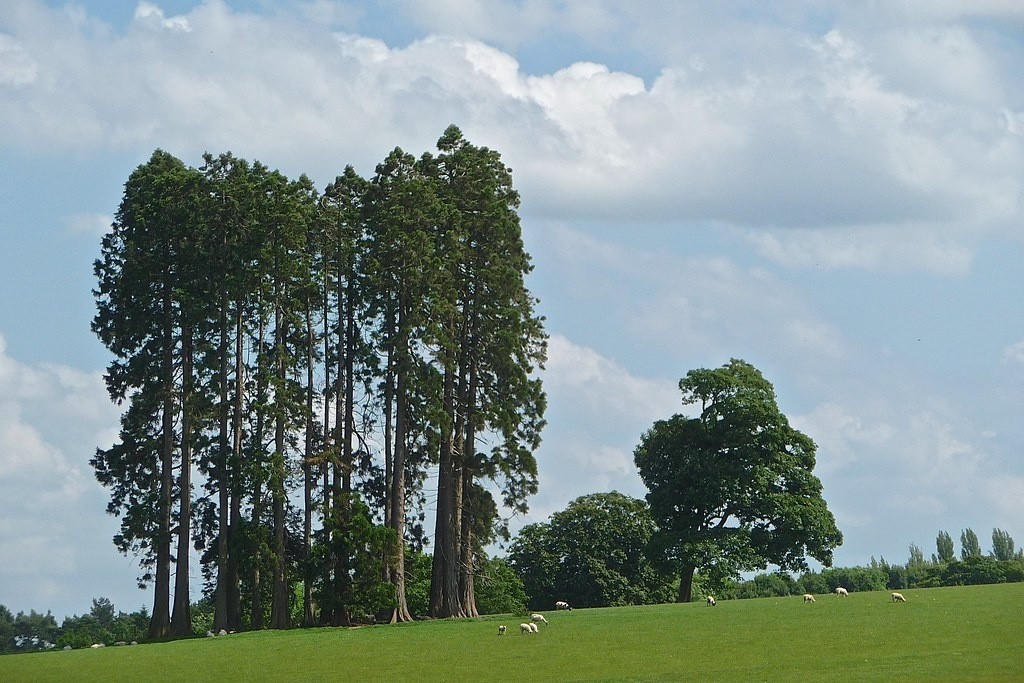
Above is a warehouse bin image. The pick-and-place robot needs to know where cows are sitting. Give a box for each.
[706,596,717,608]
[555,601,572,611]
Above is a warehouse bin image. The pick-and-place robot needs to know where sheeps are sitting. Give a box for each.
[520,624,533,635]
[63,641,138,651]
[835,587,849,598]
[499,626,506,635]
[891,592,907,604]
[803,594,816,604]
[530,623,539,633]
[531,614,549,626]
[206,629,236,638]
